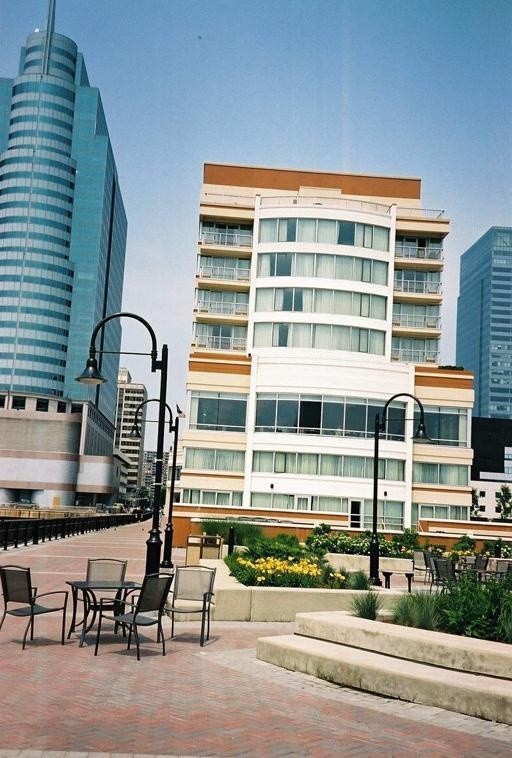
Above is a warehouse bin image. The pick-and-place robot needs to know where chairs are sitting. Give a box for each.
[413,552,511,594]
[2,560,216,660]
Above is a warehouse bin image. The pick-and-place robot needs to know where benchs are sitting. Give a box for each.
[378,569,416,592]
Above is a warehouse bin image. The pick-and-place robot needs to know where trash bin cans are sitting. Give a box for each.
[185,534,222,568]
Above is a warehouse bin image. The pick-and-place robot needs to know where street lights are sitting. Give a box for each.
[128,397,180,568]
[75,310,170,578]
[369,391,435,585]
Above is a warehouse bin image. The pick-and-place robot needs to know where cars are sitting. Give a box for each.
[8,498,39,509]
[96,502,163,516]
